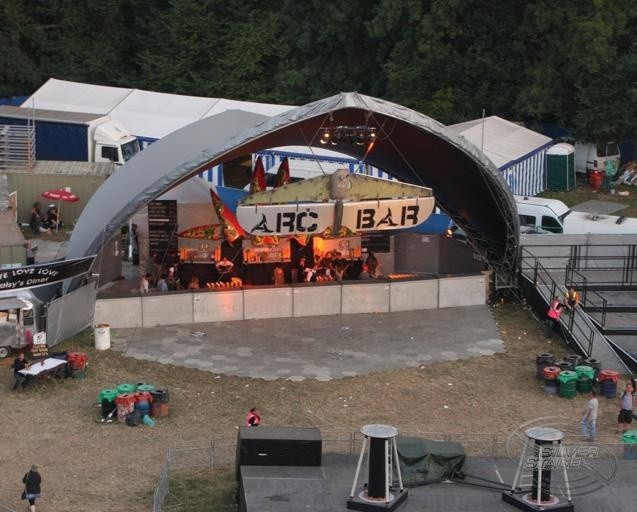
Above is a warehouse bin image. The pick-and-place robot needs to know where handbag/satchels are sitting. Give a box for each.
[22,491,26,500]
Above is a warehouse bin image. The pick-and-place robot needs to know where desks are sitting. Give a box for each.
[17,357,68,394]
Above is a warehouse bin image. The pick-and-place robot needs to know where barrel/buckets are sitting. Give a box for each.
[623,430,636,461]
[536,351,619,401]
[100,383,169,423]
[94,323,111,350]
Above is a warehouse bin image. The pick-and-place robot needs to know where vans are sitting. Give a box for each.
[512,194,571,235]
[555,134,622,180]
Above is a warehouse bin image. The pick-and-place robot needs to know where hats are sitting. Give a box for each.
[30,464,37,473]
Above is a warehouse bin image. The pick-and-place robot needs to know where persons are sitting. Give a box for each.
[543,295,562,343]
[623,168,633,185]
[246,405,260,427]
[12,352,30,392]
[135,250,235,295]
[580,390,599,442]
[563,288,580,311]
[270,245,378,286]
[29,195,62,238]
[615,383,636,435]
[22,464,41,512]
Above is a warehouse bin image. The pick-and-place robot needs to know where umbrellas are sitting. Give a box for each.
[41,189,80,234]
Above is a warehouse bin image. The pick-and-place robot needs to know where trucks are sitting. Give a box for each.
[0,104,142,169]
